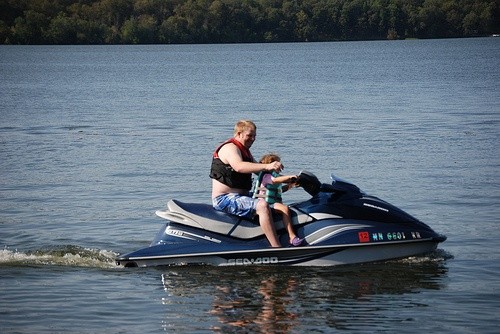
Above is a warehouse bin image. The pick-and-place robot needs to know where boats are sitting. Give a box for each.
[115,171,449,272]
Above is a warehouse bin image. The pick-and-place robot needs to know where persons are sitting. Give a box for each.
[208,120,284,248]
[253,154,304,247]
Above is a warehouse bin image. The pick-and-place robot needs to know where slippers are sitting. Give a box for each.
[289,236,304,246]
